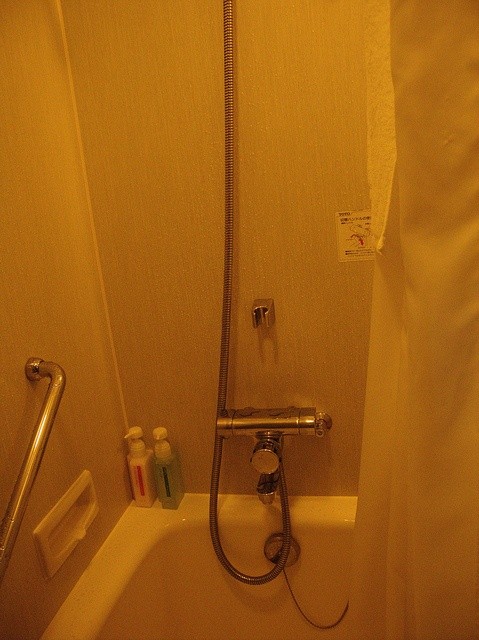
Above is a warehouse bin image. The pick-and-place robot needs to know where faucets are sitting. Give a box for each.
[250,434,284,507]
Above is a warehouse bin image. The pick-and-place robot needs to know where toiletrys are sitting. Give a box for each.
[123,427,157,509]
[150,427,184,510]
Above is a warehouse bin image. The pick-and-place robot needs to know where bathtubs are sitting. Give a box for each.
[39,494,356,638]
